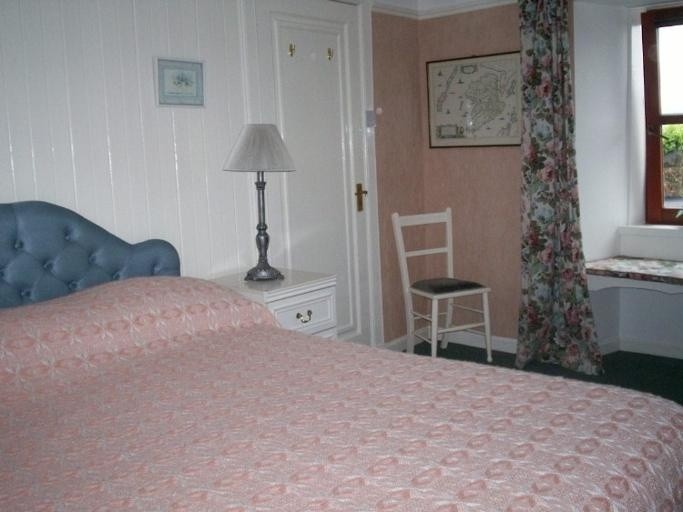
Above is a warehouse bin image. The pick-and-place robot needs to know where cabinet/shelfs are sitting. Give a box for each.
[208,269,340,347]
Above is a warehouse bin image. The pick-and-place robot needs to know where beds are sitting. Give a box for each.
[0,199,683,512]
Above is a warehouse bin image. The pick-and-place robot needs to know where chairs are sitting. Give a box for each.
[389,205,494,364]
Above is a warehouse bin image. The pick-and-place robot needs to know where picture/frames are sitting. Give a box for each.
[153,54,205,107]
[425,51,526,149]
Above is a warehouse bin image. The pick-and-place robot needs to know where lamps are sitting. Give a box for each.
[221,122,296,282]
